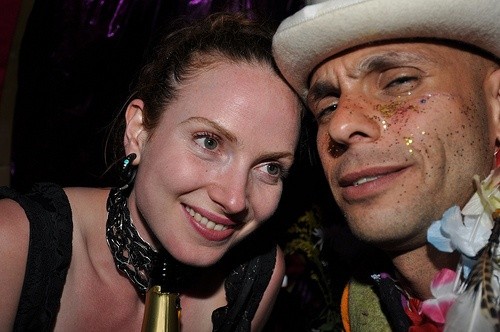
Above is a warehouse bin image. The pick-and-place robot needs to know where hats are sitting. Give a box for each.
[271,0,500,103]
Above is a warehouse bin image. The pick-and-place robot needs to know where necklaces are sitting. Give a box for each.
[370,170,500,332]
[105,180,181,304]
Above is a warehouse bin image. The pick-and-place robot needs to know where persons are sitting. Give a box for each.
[0,12,302,332]
[271,0,500,332]
